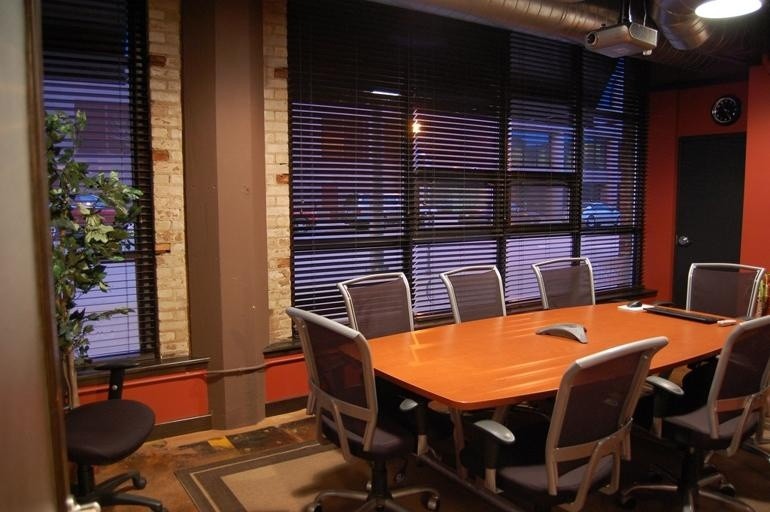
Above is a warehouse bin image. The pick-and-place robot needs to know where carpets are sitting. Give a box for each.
[175,433,489,509]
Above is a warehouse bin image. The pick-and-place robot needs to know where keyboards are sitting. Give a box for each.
[643,306,725,324]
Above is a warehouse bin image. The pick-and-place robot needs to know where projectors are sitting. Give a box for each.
[585,22,658,59]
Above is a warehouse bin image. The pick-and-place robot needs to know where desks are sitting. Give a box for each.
[369,300,744,512]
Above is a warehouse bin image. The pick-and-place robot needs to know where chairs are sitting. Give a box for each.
[643,311,770,511]
[65,360,170,510]
[440,264,505,323]
[650,262,767,318]
[531,256,599,312]
[474,336,671,511]
[340,271,414,341]
[283,298,444,512]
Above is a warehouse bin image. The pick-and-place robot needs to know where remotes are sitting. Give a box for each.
[717,319,738,327]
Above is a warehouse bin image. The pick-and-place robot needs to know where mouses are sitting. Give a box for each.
[627,301,642,307]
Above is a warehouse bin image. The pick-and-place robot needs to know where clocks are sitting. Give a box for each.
[711,94,742,124]
